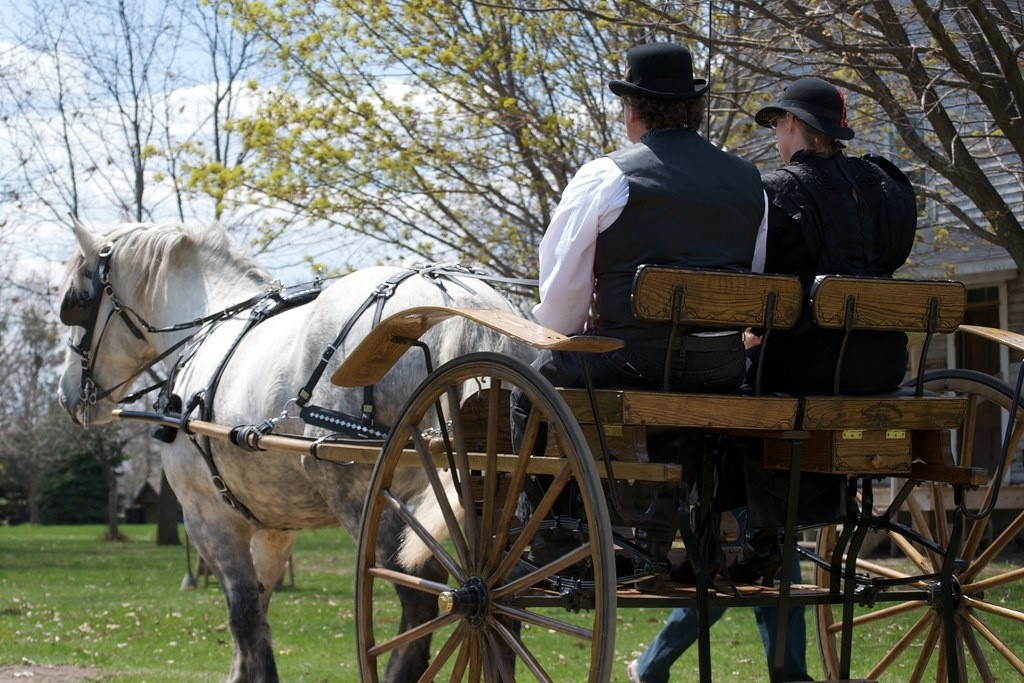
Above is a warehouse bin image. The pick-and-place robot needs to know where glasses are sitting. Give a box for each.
[768,115,780,130]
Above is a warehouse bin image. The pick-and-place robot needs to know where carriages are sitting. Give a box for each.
[57,213,1024,682]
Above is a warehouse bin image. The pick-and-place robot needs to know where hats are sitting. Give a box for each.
[754,78,855,140]
[609,42,709,98]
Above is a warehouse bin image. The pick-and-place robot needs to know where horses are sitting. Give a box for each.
[49,208,539,683]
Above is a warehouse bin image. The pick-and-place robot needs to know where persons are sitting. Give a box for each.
[668,75,910,586]
[627,544,817,683]
[508,44,770,593]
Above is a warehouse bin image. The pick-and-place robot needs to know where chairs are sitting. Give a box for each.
[330,262,987,484]
